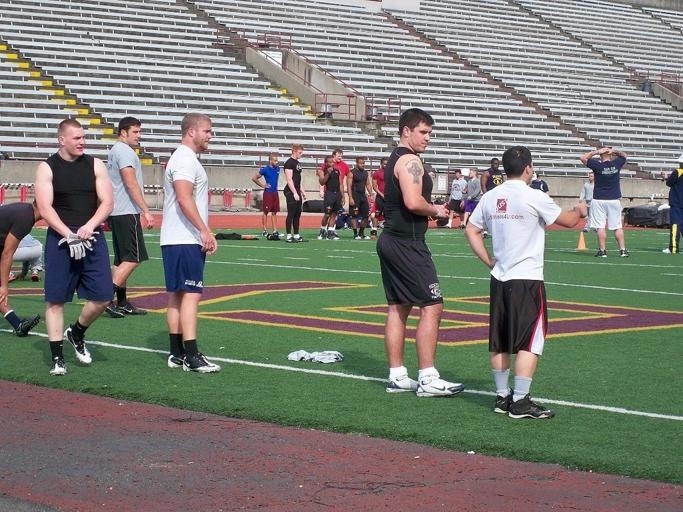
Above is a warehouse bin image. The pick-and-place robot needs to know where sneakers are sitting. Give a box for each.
[353,235,361,239]
[116,303,147,315]
[49,357,67,375]
[318,231,324,239]
[483,230,487,238]
[583,226,589,231]
[446,223,451,228]
[183,352,221,373]
[63,327,93,364]
[286,237,293,242]
[167,355,183,368]
[494,388,513,414]
[359,235,370,239]
[294,237,309,242]
[15,313,40,336]
[370,235,376,240]
[663,248,678,254]
[385,374,420,392]
[8,272,16,282]
[31,270,40,281]
[509,393,554,420]
[416,376,464,397]
[594,248,607,257]
[262,231,267,236]
[621,249,628,256]
[104,302,125,318]
[326,233,340,240]
[273,232,279,237]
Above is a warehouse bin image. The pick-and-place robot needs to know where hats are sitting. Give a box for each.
[676,155,683,163]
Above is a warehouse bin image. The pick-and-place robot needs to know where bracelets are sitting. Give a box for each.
[433,206,441,217]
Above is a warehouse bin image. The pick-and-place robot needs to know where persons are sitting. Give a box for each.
[349,193,374,228]
[317,155,345,240]
[479,158,505,239]
[282,143,307,244]
[34,119,114,374]
[370,156,388,239]
[252,153,284,238]
[103,116,154,319]
[660,155,683,253]
[0,197,42,337]
[443,170,467,229]
[376,108,465,398]
[529,173,549,195]
[368,195,386,230]
[578,146,630,258]
[335,197,353,231]
[462,145,590,420]
[578,172,597,232]
[159,113,222,373]
[8,232,44,282]
[315,149,350,236]
[459,167,482,228]
[345,157,375,240]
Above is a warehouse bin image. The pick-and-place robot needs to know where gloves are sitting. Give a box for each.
[82,231,100,253]
[67,234,86,260]
